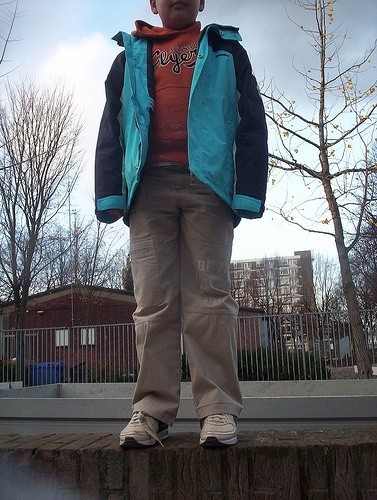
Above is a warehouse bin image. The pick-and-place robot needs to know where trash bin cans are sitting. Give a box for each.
[26,361,65,386]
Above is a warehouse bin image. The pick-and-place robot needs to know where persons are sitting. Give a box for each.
[92,0,271,447]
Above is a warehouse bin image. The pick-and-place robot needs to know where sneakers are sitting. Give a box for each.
[118,411,168,449]
[198,413,238,446]
